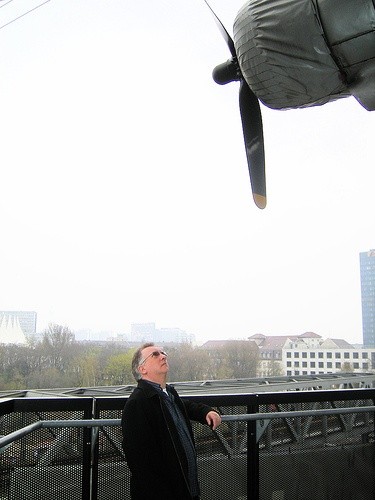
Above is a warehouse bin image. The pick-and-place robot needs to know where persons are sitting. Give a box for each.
[121,341,223,500]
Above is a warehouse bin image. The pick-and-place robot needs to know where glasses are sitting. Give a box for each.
[141,351,168,365]
